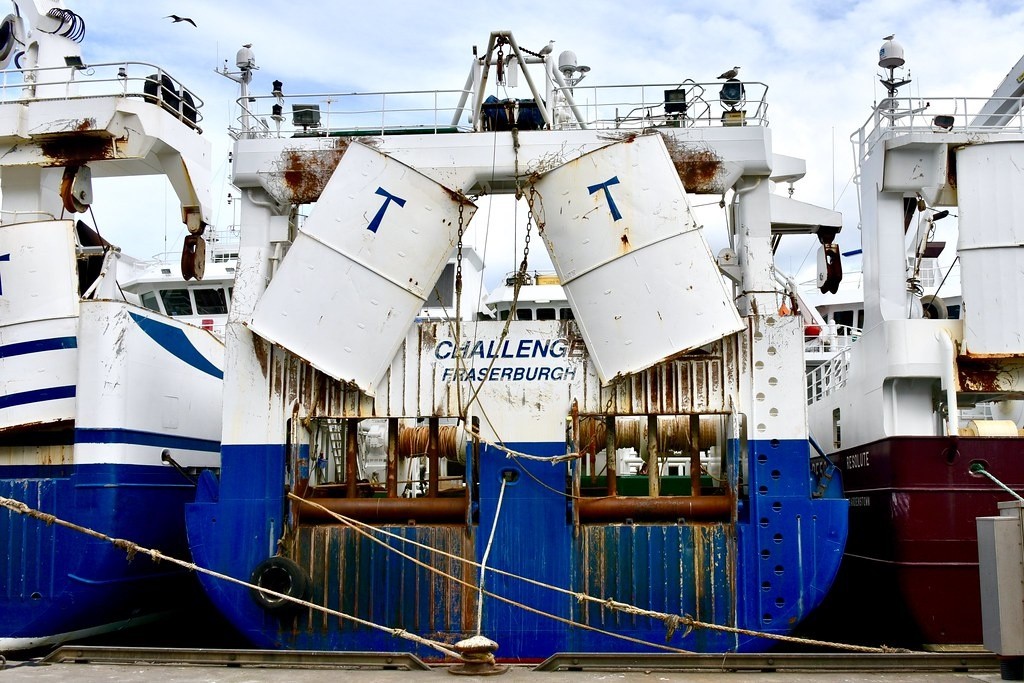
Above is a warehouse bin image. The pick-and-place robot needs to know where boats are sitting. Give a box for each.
[181,30,850,654]
[0,0,239,652]
[806,31,1024,651]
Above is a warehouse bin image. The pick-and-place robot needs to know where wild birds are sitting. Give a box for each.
[162,14,197,28]
